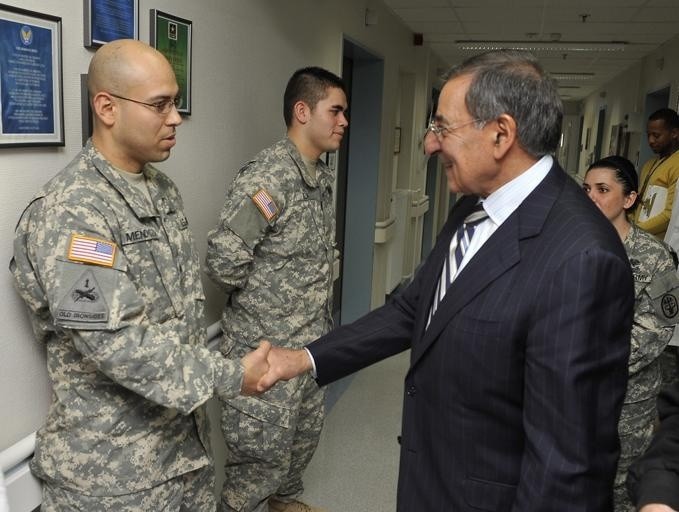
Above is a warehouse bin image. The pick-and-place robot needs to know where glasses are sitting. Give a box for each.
[429,119,480,142]
[110,94,182,113]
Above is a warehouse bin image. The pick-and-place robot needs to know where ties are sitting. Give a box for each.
[425,201,489,330]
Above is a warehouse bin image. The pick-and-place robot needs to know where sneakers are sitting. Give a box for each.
[269,499,311,512]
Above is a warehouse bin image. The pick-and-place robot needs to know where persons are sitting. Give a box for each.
[203,64,351,512]
[255,45,635,512]
[626,380,679,512]
[583,153,679,512]
[5,37,270,512]
[628,108,678,241]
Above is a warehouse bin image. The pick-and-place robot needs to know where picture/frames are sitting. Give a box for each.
[80,73,93,146]
[150,8,191,115]
[83,0,138,48]
[0,4,66,148]
